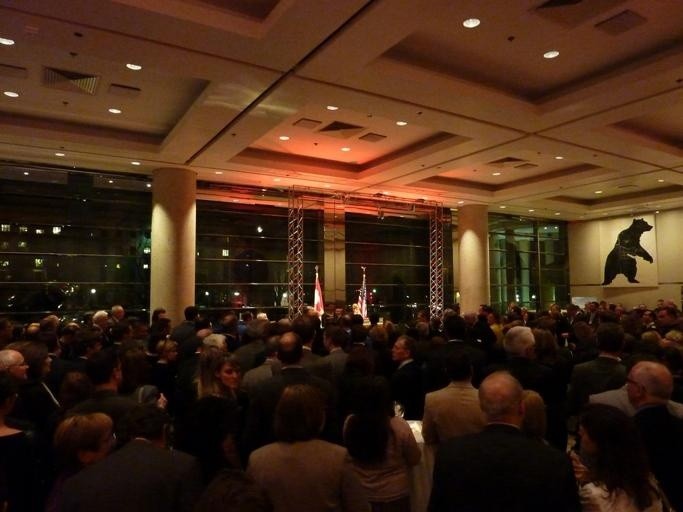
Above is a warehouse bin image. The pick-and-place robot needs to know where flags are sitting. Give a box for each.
[314,271,325,316]
[359,274,368,319]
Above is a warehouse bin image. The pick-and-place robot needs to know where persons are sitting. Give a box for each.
[0,302,683,512]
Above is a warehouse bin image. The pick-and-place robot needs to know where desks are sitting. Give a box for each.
[394,419,441,511]
